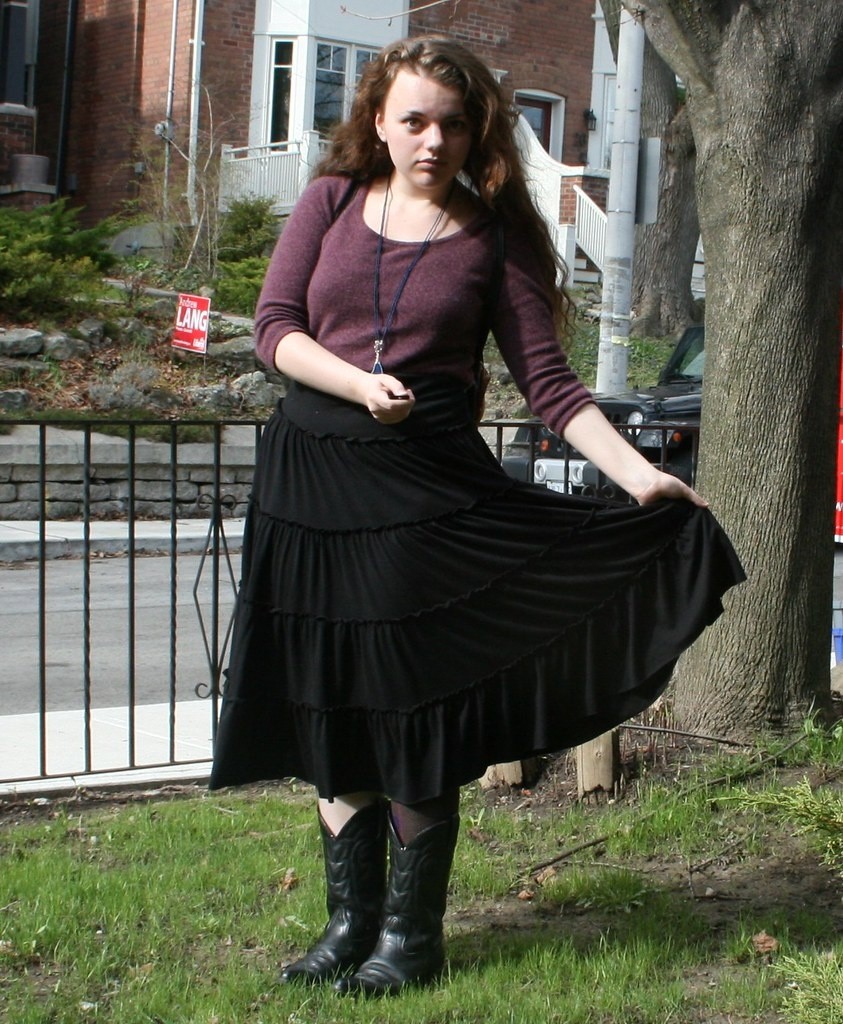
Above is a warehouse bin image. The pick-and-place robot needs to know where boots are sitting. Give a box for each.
[279,802,461,998]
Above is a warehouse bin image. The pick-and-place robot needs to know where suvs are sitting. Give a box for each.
[502,327,705,499]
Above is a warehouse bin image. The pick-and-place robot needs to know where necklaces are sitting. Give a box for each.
[371,167,455,374]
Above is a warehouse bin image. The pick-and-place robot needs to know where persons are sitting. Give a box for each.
[208,33,709,994]
